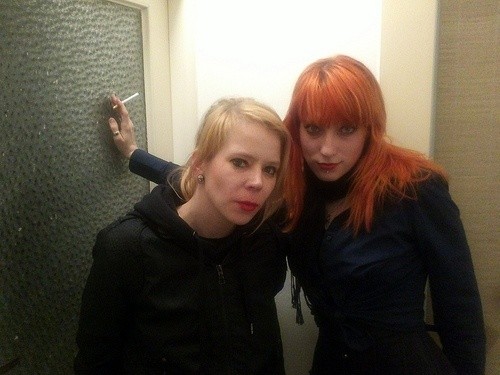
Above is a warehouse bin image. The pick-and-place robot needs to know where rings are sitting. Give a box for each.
[113,130,120,137]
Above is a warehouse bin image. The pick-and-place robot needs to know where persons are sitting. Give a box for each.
[108,55,487,375]
[73,99,292,375]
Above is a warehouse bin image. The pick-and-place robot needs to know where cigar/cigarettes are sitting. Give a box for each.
[113,91,140,108]
